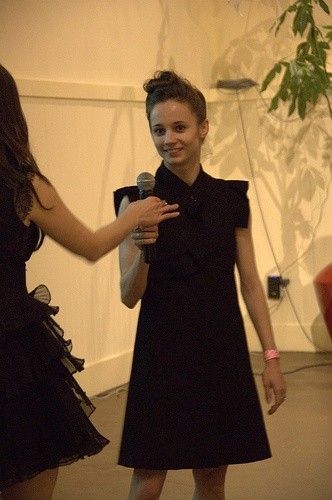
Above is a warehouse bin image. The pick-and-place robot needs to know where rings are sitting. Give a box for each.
[280,396,286,400]
[160,200,166,206]
[135,226,141,232]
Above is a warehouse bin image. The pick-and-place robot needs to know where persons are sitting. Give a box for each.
[112,71,287,500]
[0,65,180,500]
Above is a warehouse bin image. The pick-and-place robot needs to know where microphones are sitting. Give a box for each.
[136,171,156,263]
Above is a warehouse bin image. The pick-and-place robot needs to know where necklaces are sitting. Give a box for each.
[169,166,200,186]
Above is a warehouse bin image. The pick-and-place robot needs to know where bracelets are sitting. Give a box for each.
[263,349,283,362]
